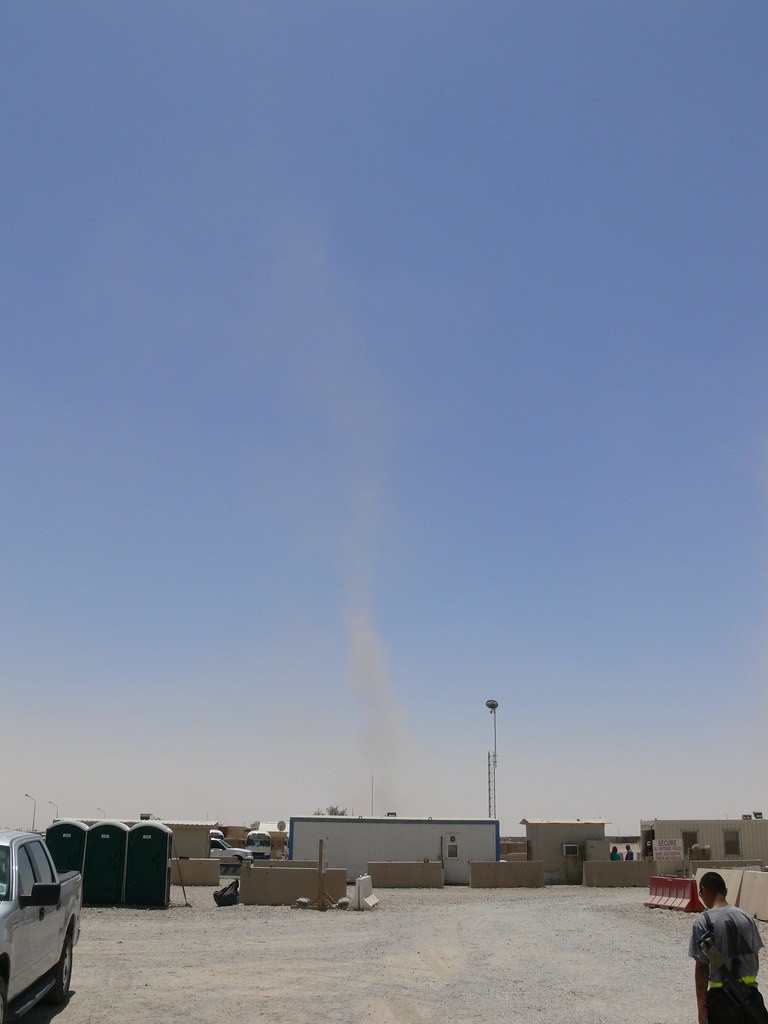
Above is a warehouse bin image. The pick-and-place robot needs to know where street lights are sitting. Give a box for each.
[25,794,36,831]
[49,801,58,818]
[486,700,498,820]
[98,808,105,819]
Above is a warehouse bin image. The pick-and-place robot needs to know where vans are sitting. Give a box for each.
[209,829,223,840]
[243,830,274,858]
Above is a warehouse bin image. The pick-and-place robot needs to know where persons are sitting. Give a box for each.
[283,842,289,859]
[610,846,622,861]
[624,845,633,860]
[688,872,764,1024]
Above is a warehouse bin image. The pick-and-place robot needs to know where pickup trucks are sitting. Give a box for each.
[0,831,83,1024]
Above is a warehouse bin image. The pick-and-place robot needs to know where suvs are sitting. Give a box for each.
[210,838,254,865]
